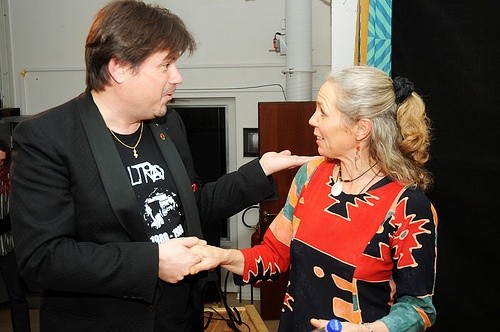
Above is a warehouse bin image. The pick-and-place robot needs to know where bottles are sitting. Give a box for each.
[308,319,342,332]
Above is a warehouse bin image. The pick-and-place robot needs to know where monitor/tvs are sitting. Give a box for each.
[243,128,259,157]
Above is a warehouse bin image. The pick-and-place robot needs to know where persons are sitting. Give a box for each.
[0,1,320,332]
[188,66,438,332]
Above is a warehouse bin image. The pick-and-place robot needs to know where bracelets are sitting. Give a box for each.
[362,322,374,331]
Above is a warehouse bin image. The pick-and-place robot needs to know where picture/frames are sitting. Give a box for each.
[242,127,260,156]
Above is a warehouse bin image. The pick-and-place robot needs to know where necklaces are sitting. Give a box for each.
[331,161,378,197]
[108,121,144,159]
